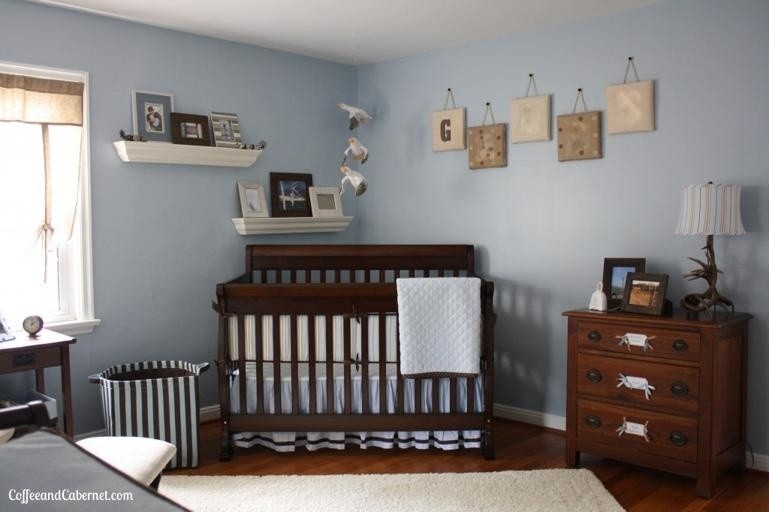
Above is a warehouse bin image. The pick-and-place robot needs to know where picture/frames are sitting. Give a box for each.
[132,90,241,148]
[238,171,342,218]
[0,315,16,342]
[603,258,645,305]
[622,271,669,316]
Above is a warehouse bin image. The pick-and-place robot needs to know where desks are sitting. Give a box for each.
[0,328,77,437]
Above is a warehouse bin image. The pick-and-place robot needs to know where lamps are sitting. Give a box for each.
[675,180,746,319]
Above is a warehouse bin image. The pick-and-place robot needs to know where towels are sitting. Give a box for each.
[396,278,483,375]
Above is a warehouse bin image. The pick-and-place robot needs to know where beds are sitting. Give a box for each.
[213,245,497,462]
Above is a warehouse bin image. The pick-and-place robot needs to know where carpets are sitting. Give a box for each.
[156,467,628,512]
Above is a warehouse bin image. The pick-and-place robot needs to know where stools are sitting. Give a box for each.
[75,436,178,490]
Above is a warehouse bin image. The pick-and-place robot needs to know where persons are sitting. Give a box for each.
[147,106,161,130]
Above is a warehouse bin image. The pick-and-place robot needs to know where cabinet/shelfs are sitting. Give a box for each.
[562,308,755,499]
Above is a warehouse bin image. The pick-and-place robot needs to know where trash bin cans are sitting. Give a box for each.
[88,361,210,469]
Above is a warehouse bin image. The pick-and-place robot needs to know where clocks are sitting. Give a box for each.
[23,315,43,335]
